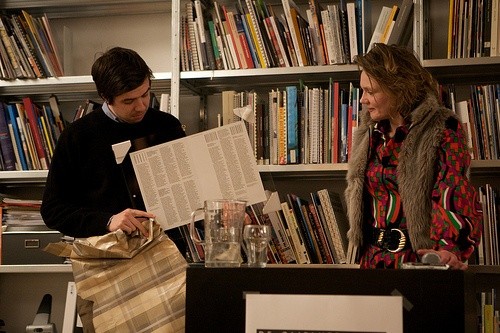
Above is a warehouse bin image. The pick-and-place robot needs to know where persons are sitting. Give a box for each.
[346,42,484,270]
[41,46,252,258]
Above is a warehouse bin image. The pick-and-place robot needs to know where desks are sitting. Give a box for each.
[184,264,466,333]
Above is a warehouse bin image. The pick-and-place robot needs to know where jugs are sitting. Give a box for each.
[190,199,247,268]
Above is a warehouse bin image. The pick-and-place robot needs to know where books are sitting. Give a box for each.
[0,10,171,231]
[180,0,500,333]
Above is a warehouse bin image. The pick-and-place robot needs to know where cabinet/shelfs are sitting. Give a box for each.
[0,0,500,333]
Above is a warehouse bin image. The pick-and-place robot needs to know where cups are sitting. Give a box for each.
[246,226,268,267]
